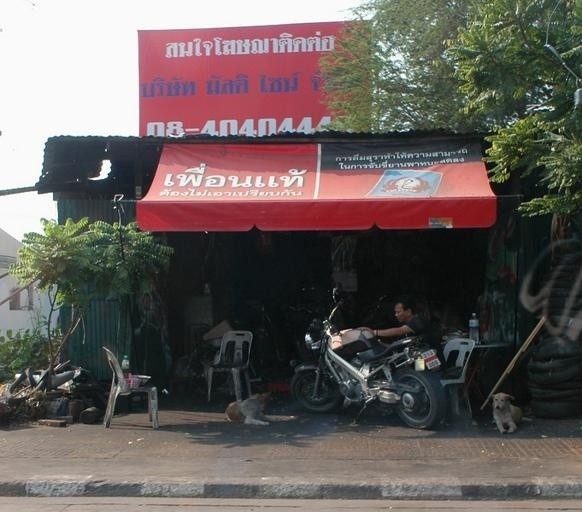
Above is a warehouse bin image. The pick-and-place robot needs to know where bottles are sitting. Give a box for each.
[467,311,480,344]
[120,354,130,373]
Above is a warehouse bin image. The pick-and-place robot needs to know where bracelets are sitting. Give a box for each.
[374,329,378,337]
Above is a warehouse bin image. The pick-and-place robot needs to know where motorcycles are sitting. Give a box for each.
[291,286,445,429]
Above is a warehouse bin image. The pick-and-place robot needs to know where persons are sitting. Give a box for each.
[372,294,447,369]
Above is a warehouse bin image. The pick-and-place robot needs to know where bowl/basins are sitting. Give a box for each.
[134,374,151,386]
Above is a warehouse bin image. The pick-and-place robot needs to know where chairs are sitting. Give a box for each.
[439,338,475,421]
[100,345,159,431]
[205,330,254,402]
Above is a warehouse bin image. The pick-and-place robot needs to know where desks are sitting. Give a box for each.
[445,338,509,408]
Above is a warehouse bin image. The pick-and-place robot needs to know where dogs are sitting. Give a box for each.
[224,390,277,427]
[491,392,523,433]
